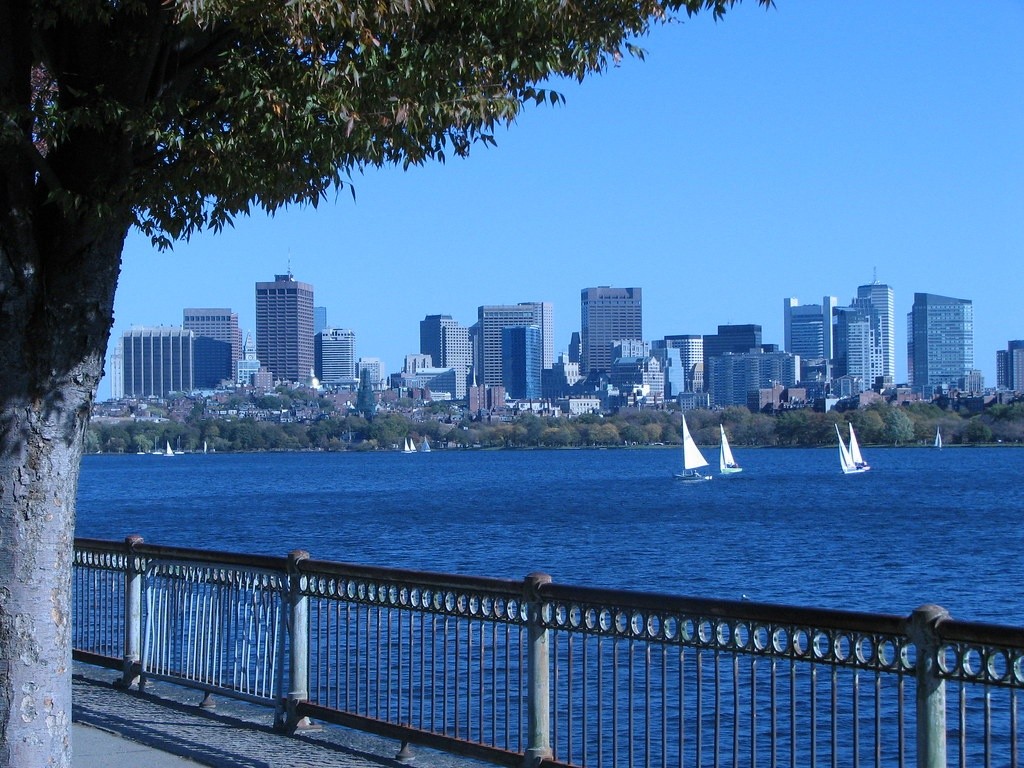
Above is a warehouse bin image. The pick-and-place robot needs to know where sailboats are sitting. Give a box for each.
[401,436,430,455]
[718,422,742,475]
[835,420,872,476]
[136,437,216,457]
[674,414,710,484]
